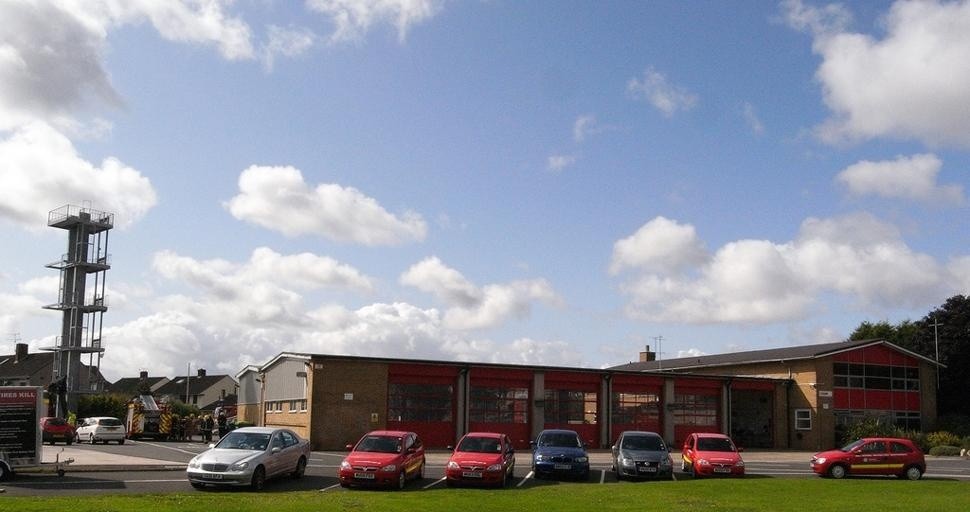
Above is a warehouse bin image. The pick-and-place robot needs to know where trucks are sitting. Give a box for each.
[0,386,42,482]
[16,420,19,422]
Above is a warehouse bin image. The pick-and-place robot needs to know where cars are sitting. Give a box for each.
[187,426,310,492]
[809,437,926,480]
[682,433,746,479]
[530,428,591,481]
[614,431,674,479]
[337,431,427,492]
[41,417,126,444]
[445,433,515,487]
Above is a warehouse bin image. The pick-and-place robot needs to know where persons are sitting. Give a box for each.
[217,411,227,439]
[168,413,213,444]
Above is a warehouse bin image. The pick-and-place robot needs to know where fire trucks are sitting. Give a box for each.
[127,398,173,440]
